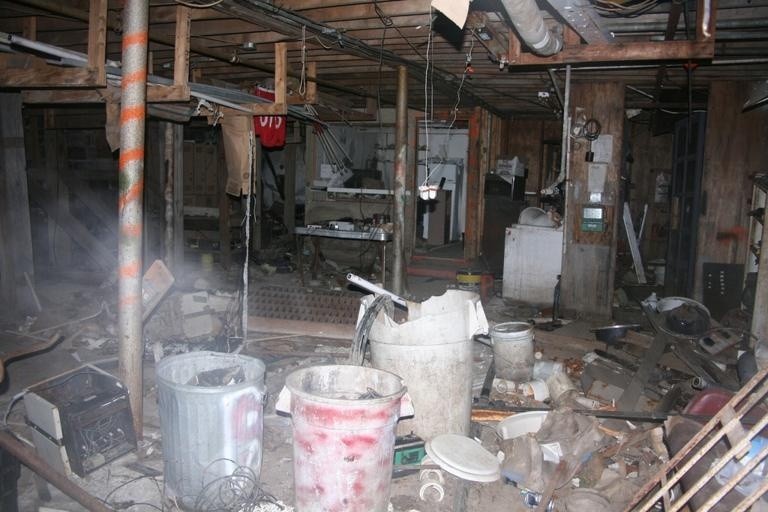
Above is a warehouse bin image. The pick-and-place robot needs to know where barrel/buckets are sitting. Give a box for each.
[285,365,408,512]
[155,351,267,511]
[369,313,477,441]
[490,322,536,384]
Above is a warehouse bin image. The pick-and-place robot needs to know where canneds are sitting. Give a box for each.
[524,491,554,512]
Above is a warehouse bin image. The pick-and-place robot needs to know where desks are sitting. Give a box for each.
[294,221,393,283]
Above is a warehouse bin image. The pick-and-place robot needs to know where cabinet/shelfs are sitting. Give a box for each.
[739,173,767,348]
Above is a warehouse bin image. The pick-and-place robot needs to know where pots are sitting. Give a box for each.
[588,324,643,345]
